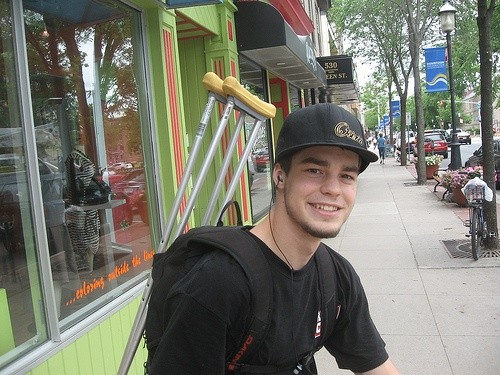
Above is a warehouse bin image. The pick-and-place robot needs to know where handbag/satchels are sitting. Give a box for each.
[68,172,112,204]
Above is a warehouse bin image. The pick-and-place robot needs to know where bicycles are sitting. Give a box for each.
[457,171,497,261]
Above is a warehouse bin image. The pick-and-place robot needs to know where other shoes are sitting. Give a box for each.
[380,161,382,164]
[383,163,384,164]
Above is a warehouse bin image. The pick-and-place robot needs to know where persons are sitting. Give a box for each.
[148,105,398,375]
[31,129,101,292]
[374,134,387,164]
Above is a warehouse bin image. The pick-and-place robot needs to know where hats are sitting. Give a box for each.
[276,103,379,174]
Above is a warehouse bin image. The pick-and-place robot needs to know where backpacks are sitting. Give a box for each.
[146,200,337,375]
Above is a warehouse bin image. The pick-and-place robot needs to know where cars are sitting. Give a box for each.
[251,147,271,172]
[0,128,147,256]
[464,137,500,190]
[446,128,471,145]
[386,128,449,159]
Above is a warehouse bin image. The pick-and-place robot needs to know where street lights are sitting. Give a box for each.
[436,0,463,171]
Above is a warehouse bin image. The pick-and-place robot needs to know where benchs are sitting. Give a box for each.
[432,169,450,199]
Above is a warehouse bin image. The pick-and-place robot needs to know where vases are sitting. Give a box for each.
[138,201,147,223]
[453,187,469,207]
[414,163,439,178]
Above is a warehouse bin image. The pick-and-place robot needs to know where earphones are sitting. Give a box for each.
[276,175,284,183]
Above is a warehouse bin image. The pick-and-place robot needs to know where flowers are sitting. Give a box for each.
[442,166,498,188]
[410,154,444,166]
[136,189,147,202]
